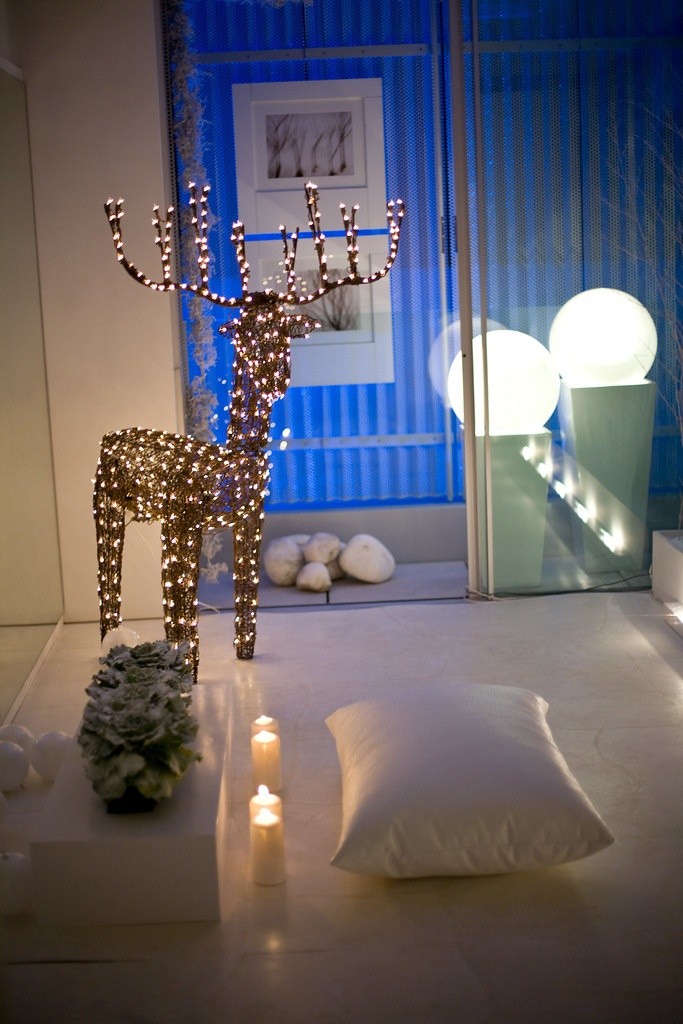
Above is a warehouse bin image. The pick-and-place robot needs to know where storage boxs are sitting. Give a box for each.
[29,679,232,930]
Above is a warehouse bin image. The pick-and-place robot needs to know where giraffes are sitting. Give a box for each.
[92,180,408,685]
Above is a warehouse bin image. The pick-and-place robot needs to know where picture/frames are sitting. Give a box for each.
[232,78,388,235]
[244,235,395,388]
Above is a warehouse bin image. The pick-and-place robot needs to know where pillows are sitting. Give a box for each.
[323,687,615,880]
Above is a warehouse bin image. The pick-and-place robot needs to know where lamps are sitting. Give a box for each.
[448,330,560,589]
[549,286,658,573]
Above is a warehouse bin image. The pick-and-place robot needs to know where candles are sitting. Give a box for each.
[252,730,282,793]
[250,809,287,886]
[249,784,282,819]
[252,714,279,736]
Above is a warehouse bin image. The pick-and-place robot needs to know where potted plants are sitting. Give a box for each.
[78,637,203,815]
